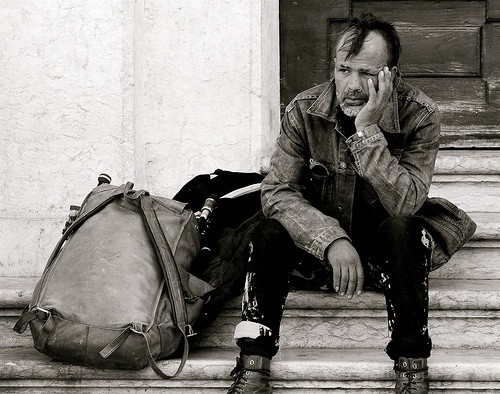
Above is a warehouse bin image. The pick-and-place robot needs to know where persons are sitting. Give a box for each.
[225,13,445,394]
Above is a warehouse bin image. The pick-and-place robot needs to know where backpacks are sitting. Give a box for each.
[14,173,216,380]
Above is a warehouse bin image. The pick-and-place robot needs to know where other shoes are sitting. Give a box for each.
[227,354,272,393]
[394,357,429,394]
